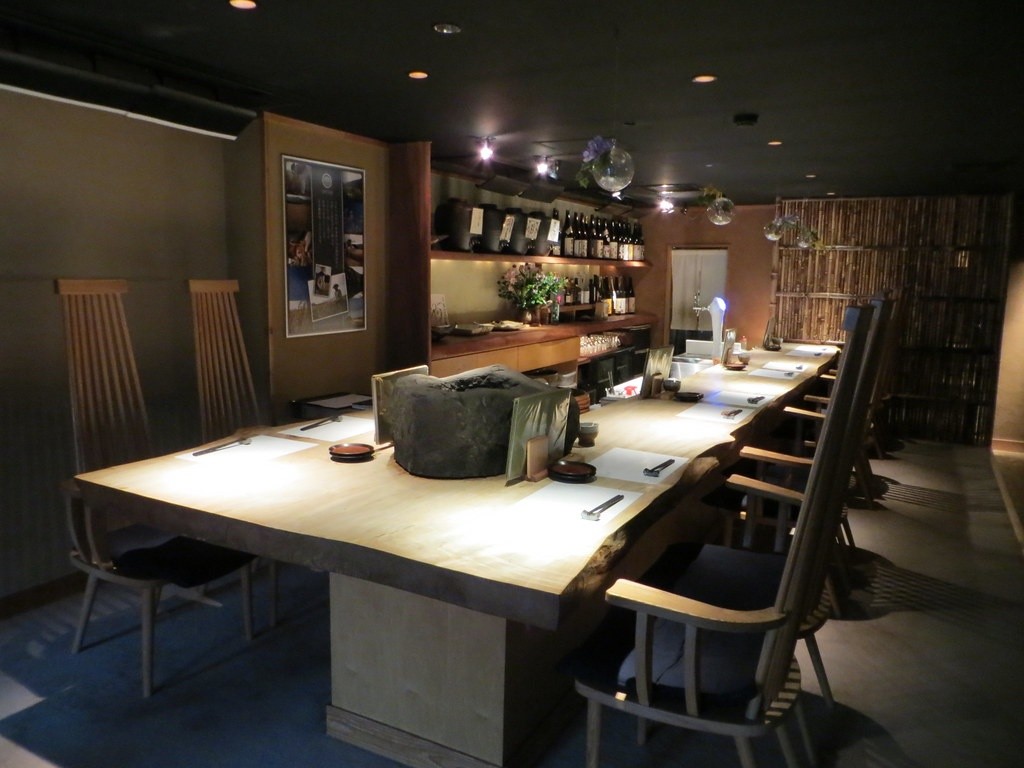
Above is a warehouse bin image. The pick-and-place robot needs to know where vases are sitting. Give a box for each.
[518,305,532,325]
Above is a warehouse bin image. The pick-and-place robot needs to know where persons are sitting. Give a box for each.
[316,266,331,296]
[333,284,343,308]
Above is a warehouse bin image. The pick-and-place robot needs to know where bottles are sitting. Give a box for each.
[551,208,645,261]
[540,274,636,324]
[741,336,747,349]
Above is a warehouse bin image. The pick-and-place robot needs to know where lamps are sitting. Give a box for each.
[477,137,494,158]
[702,297,726,342]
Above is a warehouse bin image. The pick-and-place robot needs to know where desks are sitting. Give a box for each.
[77,341,845,766]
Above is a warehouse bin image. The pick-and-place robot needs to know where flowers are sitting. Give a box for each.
[499,264,560,306]
[574,135,615,189]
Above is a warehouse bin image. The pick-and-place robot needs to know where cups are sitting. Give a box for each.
[577,423,599,446]
[580,334,619,356]
[738,353,750,365]
[772,337,783,345]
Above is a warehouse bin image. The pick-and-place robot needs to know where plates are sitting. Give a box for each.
[492,322,524,330]
[726,364,744,371]
[453,322,495,335]
[547,459,597,480]
[766,344,780,350]
[328,443,376,460]
[674,391,703,402]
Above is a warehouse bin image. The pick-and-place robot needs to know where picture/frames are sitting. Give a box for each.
[283,152,368,340]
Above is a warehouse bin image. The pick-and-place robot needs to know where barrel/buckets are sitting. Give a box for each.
[433,197,553,256]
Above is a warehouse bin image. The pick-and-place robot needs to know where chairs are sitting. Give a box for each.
[568,286,914,768]
[186,277,287,642]
[52,277,238,696]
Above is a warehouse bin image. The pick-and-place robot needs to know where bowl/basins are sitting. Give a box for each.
[663,378,681,393]
[432,326,453,341]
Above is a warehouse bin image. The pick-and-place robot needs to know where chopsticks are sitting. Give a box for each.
[650,458,675,473]
[300,412,342,431]
[192,434,259,455]
[588,494,623,514]
